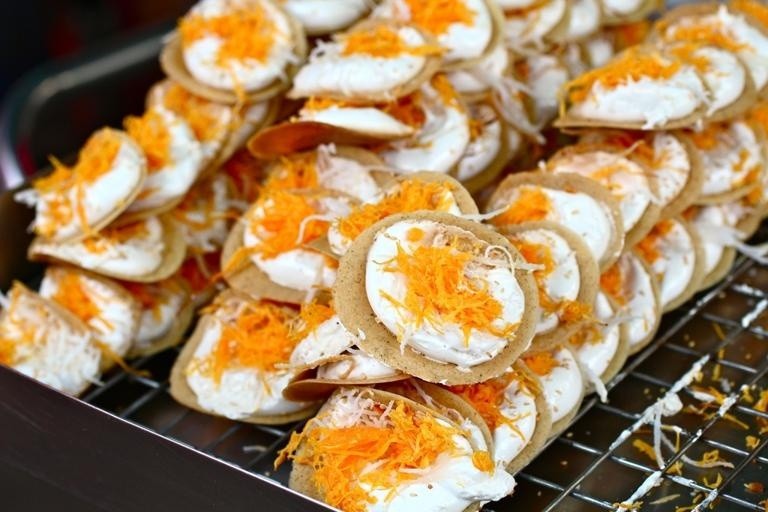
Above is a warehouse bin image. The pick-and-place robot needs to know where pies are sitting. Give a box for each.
[0,1,768,511]
